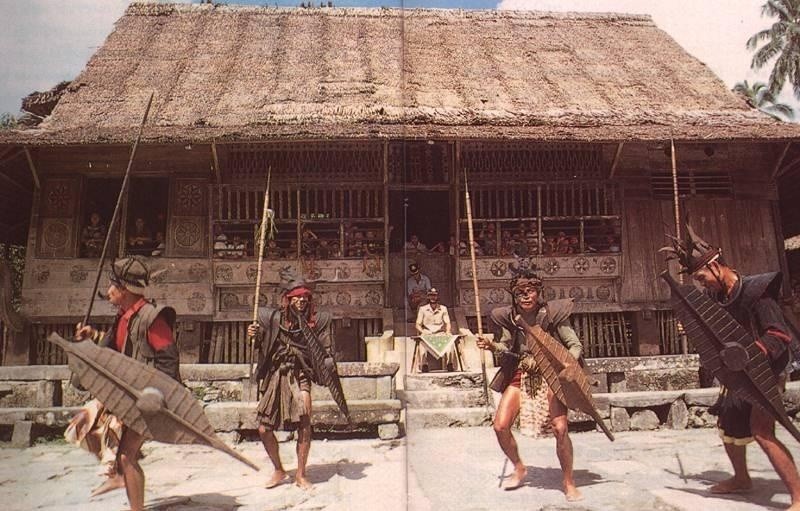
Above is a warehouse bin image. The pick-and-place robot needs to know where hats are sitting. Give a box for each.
[409,263,420,277]
[427,286,439,295]
[285,280,312,299]
[506,253,544,291]
[663,213,721,275]
[110,256,150,296]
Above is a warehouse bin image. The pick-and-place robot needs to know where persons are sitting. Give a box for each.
[63,256,181,511]
[658,214,800,511]
[79,211,165,257]
[475,260,582,501]
[407,264,431,322]
[403,222,621,256]
[213,225,384,259]
[415,287,455,372]
[248,268,338,490]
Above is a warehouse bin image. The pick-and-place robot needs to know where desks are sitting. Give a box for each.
[411,334,467,373]
